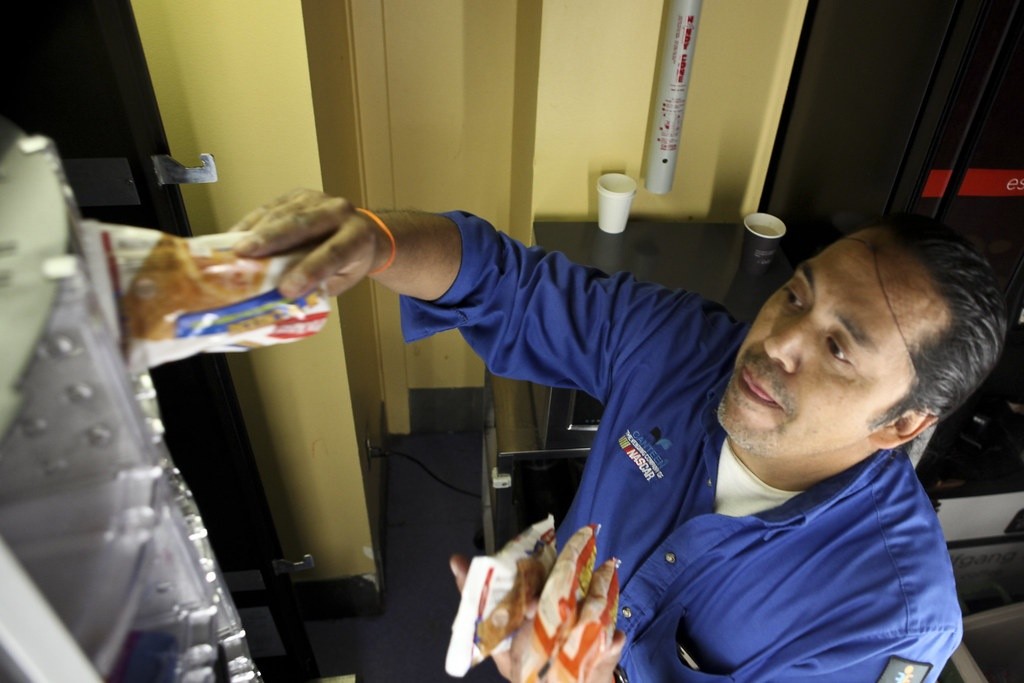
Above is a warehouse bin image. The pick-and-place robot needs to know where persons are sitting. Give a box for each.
[229,186,1007,683]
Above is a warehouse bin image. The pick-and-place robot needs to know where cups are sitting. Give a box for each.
[742,213,786,277]
[597,173,637,234]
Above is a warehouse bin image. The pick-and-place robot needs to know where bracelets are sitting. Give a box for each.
[355,207,396,276]
